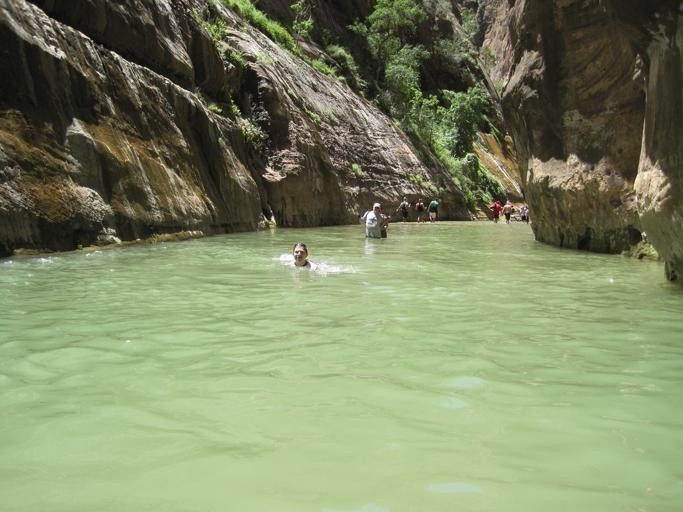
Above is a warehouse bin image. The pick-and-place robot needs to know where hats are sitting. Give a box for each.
[373,203,381,209]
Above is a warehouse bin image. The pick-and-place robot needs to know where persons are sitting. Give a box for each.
[287,242,318,270]
[510,205,513,214]
[359,202,384,239]
[500,199,510,224]
[414,198,427,223]
[255,215,266,231]
[523,206,529,222]
[379,211,387,239]
[426,197,439,223]
[394,196,410,222]
[488,200,501,223]
[261,210,276,229]
[520,206,524,221]
